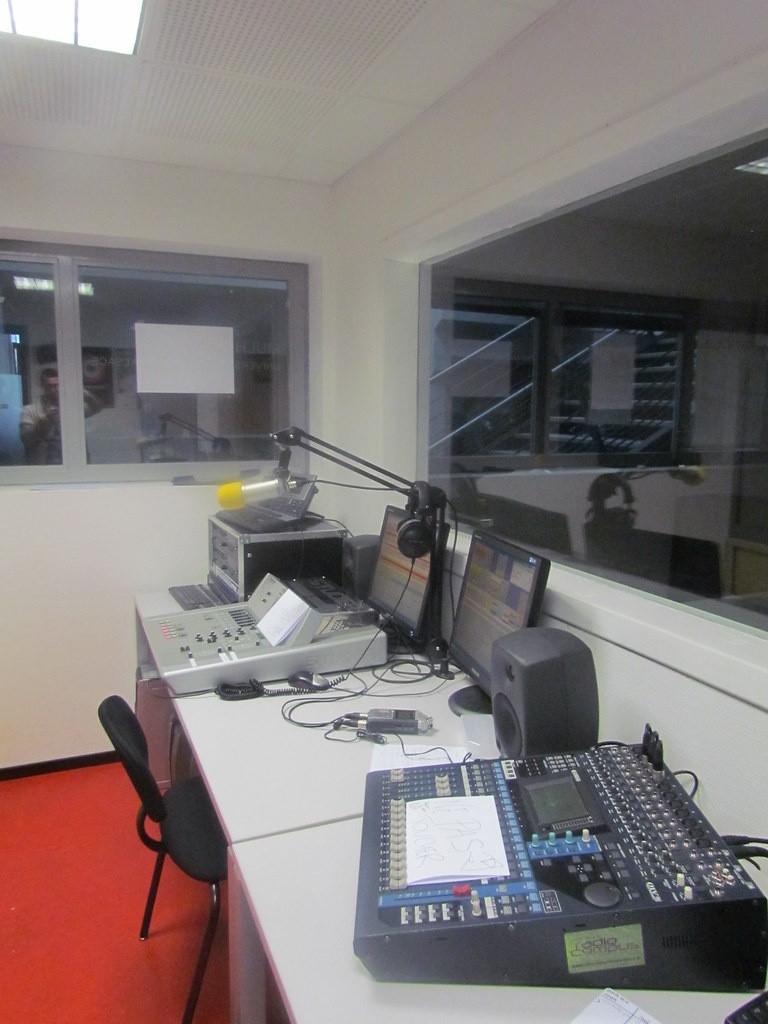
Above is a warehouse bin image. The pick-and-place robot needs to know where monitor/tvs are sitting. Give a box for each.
[462,491,571,555]
[604,528,722,600]
[368,504,451,655]
[448,527,553,716]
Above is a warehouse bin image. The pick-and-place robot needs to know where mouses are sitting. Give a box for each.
[288,670,329,689]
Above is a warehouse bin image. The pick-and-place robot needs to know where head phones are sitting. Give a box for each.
[584,474,637,550]
[449,461,497,535]
[397,480,434,559]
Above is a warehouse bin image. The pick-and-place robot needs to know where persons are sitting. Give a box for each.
[19,369,63,465]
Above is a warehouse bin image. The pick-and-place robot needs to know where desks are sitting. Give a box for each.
[129,589,768,1024]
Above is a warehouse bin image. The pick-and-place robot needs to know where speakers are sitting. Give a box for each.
[492,626,601,758]
[343,534,381,599]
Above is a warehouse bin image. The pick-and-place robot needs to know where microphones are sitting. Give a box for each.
[218,478,310,510]
[668,466,706,485]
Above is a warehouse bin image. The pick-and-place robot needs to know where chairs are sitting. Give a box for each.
[97,696,228,1023]
